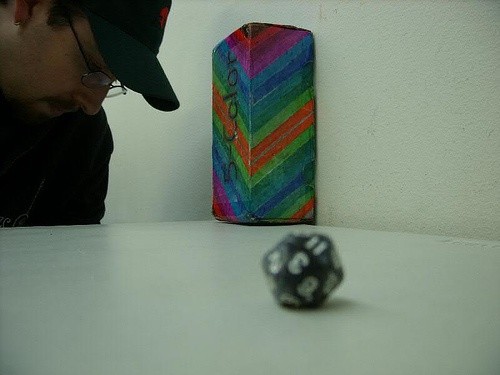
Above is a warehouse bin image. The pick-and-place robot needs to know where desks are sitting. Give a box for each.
[0,220,500,375]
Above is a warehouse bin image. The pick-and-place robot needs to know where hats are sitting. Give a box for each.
[55,0,180,112]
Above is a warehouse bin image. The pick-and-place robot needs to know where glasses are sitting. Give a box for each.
[65,14,127,95]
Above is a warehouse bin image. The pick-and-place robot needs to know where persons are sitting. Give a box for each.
[0,0,181,227]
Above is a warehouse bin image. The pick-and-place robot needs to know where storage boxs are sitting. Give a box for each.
[211,23,318,221]
